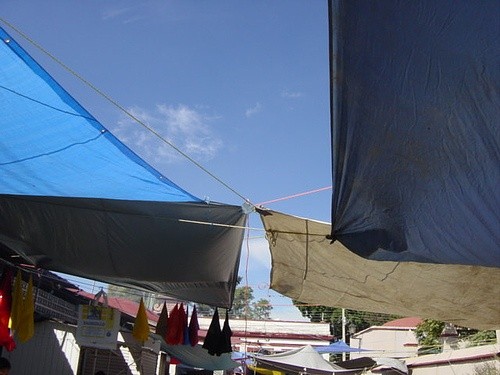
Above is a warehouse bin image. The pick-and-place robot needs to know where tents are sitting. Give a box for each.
[239,344,361,375]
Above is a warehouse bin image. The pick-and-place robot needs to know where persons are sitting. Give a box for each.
[0,357,11,375]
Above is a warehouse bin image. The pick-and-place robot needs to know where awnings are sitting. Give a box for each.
[312,340,380,355]
[0,24,208,204]
[0,193,246,309]
[254,203,499,336]
[326,1,499,269]
[63,285,252,347]
[146,330,245,372]
